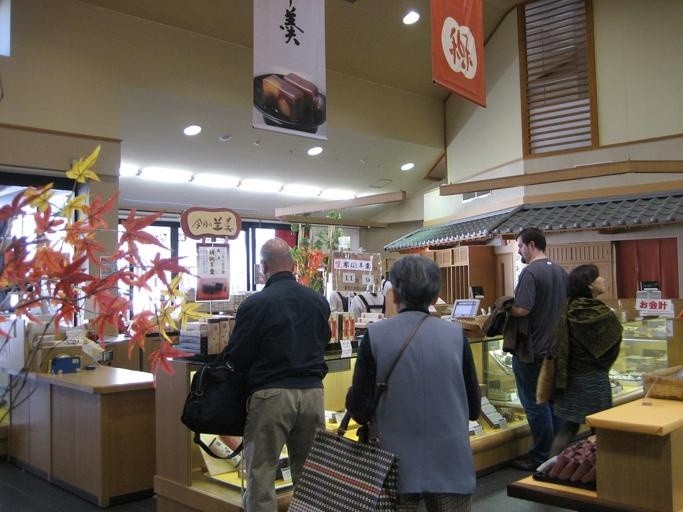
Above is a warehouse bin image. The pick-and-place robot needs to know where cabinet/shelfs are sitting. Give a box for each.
[0,295,683,512]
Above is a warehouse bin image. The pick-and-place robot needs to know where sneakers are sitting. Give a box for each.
[513,459,538,471]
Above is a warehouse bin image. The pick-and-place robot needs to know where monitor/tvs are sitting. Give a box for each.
[452,298,480,317]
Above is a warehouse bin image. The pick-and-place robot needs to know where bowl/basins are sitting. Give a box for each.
[253,72,326,135]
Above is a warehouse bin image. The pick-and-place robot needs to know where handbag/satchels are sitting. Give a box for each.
[287,410,399,512]
[536,354,555,404]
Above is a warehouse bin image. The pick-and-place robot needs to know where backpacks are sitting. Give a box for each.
[181,364,248,458]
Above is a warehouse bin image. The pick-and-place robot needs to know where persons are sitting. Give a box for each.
[545,264,624,459]
[220,237,332,512]
[502,227,568,471]
[329,275,392,323]
[345,253,481,512]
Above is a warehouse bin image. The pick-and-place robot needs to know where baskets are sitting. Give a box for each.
[642,365,683,400]
